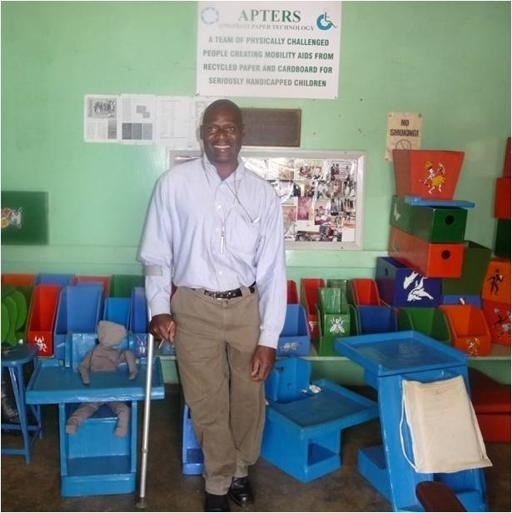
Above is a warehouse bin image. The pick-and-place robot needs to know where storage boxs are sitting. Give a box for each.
[1,135,510,441]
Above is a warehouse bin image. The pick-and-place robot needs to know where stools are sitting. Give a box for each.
[3,343,42,466]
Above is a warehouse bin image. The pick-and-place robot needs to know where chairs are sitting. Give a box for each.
[61,329,136,497]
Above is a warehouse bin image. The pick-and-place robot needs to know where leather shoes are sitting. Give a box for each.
[204,491,230,511]
[229,476,255,507]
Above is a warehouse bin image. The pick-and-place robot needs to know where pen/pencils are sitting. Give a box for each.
[158,320,174,349]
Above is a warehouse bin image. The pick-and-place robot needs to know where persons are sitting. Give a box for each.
[136,98,289,510]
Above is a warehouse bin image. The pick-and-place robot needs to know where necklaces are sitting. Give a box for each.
[205,163,256,255]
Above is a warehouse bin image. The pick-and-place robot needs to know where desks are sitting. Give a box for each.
[21,355,167,499]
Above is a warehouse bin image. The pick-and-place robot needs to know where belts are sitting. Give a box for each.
[191,281,256,299]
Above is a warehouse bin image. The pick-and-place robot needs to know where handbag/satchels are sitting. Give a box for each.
[397,375,493,474]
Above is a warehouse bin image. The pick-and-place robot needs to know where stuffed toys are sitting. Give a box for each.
[54,318,141,443]
